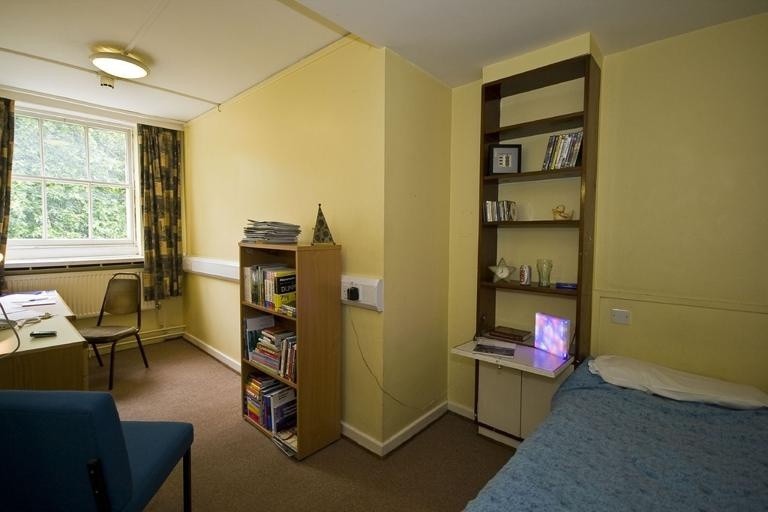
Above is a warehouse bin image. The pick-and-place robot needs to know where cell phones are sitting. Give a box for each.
[30,331,56,338]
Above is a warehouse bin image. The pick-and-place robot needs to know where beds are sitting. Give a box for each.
[459,355,768,512]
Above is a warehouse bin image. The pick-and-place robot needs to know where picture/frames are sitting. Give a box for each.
[488,144,522,175]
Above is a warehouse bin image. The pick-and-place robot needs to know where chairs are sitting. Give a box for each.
[0,389,195,512]
[76,272,149,391]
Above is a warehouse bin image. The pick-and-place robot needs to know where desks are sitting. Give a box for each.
[0,290,77,324]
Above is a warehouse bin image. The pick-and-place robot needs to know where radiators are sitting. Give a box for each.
[3,267,162,320]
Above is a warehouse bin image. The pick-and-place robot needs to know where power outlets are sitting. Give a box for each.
[341,285,361,302]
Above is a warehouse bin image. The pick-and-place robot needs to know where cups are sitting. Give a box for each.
[497,201,517,222]
[483,200,497,222]
[537,257,553,288]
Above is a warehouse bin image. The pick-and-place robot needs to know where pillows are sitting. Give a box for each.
[586,354,768,412]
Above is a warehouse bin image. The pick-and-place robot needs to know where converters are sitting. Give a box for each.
[347,287,359,301]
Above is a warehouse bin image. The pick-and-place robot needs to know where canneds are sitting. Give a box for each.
[520,264,531,285]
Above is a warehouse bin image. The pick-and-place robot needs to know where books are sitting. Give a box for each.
[242,218,301,244]
[491,325,532,342]
[241,264,299,458]
[542,131,584,170]
[482,199,517,223]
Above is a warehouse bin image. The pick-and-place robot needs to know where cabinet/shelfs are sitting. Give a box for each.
[0,316,90,391]
[475,54,602,369]
[474,356,575,451]
[238,241,343,460]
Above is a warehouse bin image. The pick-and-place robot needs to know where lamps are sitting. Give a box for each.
[90,53,150,80]
